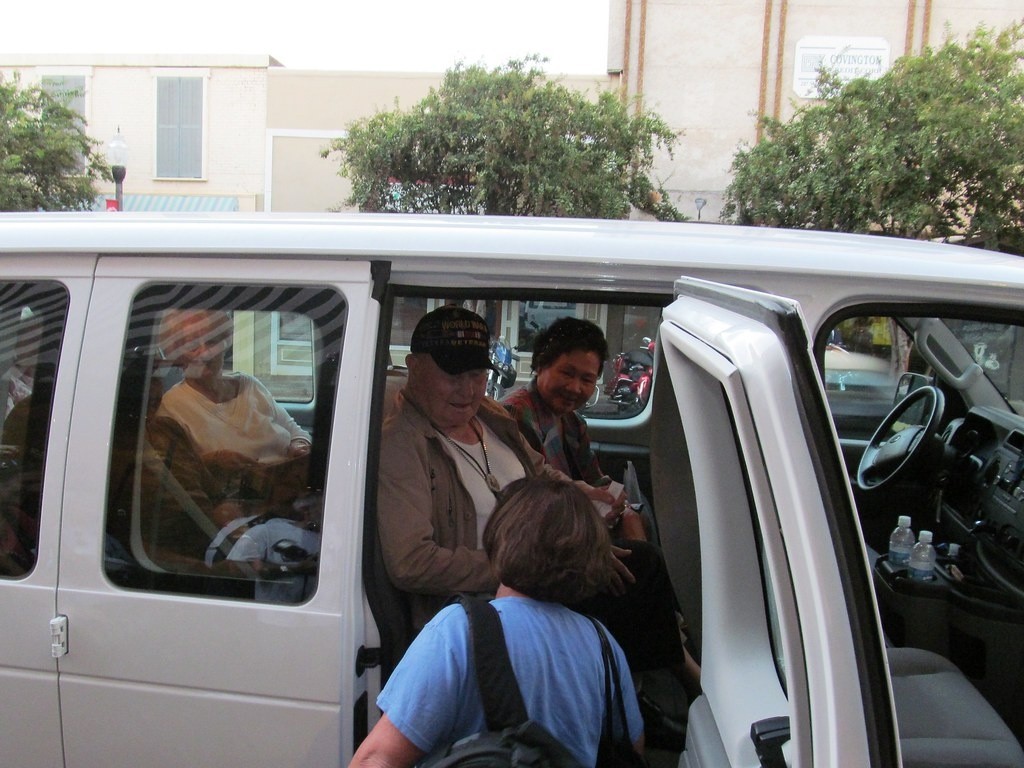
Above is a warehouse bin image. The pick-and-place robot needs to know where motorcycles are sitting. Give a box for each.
[604,335,656,418]
[485,335,521,402]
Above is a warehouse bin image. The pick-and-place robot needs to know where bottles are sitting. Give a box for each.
[889,515,915,567]
[908,530,937,583]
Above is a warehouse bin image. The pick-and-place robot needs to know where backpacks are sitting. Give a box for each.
[413,593,584,768]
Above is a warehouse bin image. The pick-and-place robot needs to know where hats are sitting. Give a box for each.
[411,303,501,377]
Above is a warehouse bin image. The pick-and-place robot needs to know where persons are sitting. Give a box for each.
[205,501,258,568]
[905,340,932,384]
[498,318,701,684]
[0,312,63,519]
[155,309,318,522]
[372,303,688,749]
[225,494,323,604]
[347,477,645,768]
[142,361,260,576]
[825,328,843,389]
[840,317,877,399]
[0,460,38,576]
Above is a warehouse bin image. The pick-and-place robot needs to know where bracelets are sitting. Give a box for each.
[296,440,310,454]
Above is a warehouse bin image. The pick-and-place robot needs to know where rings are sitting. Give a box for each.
[621,504,626,511]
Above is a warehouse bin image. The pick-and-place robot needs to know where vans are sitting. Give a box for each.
[0,208,1024,768]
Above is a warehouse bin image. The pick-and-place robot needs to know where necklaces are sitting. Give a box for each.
[216,402,222,411]
[422,411,501,492]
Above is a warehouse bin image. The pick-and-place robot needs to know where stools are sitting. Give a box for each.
[890,649,1024,768]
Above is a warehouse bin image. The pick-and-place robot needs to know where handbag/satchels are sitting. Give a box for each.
[584,614,646,768]
